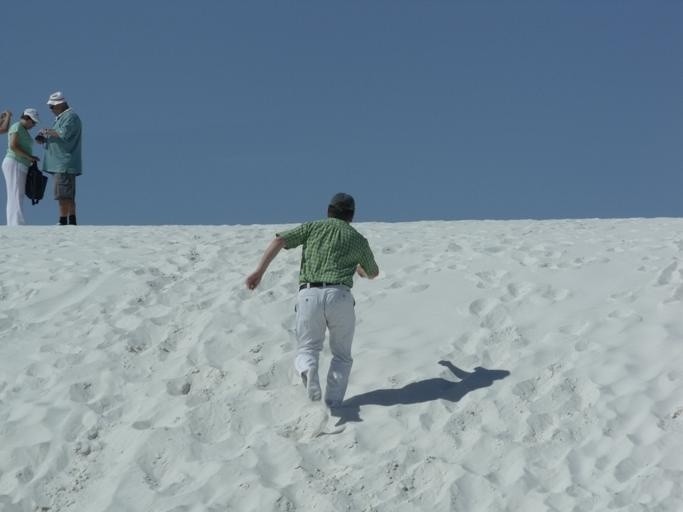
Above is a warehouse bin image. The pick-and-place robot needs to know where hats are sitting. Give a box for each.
[47,92,65,105]
[24,109,39,123]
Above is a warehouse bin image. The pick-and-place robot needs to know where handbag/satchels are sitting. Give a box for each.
[26,161,48,204]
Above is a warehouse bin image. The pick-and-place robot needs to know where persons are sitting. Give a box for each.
[1,107,41,226]
[35,89,84,224]
[244,190,381,411]
[0,109,13,135]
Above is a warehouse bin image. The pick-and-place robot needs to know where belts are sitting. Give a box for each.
[299,282,344,290]
